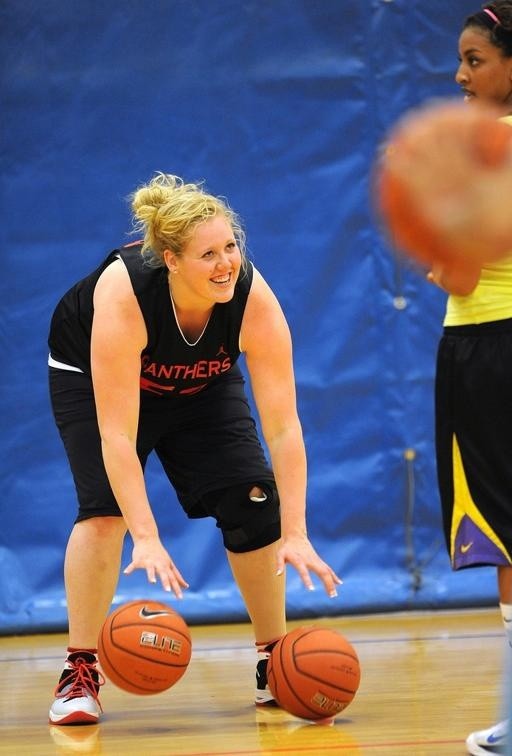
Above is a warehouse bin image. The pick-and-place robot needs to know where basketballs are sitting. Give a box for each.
[97,600,191,696]
[374,100,511,264]
[267,626,361,719]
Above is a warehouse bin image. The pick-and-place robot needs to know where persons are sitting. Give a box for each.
[45,170,347,730]
[422,3,511,756]
[380,111,511,298]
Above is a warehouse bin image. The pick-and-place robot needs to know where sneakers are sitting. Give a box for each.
[465,714,512,755]
[253,638,285,708]
[46,649,100,725]
